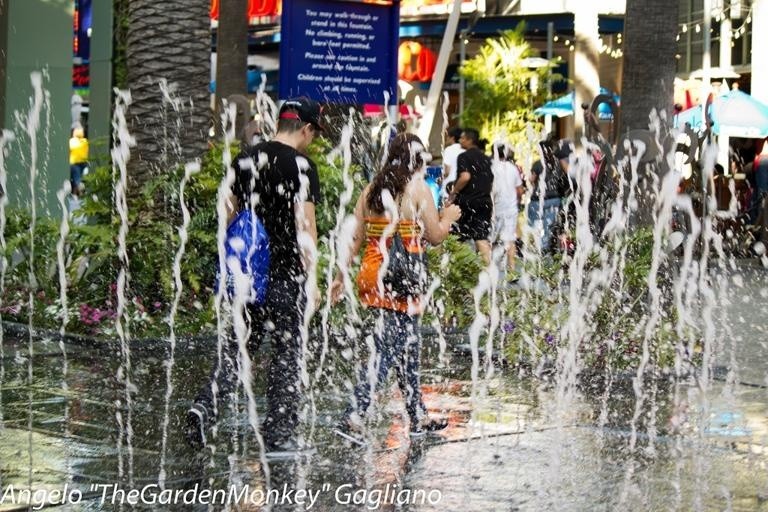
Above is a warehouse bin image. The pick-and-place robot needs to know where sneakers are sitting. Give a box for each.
[261,430,310,451]
[184,403,210,457]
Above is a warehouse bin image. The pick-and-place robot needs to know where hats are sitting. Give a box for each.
[280,95,327,131]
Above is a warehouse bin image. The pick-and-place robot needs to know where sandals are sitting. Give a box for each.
[331,418,368,447]
[410,419,448,437]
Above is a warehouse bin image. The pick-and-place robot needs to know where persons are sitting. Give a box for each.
[181,94,326,455]
[431,115,768,287]
[323,129,461,450]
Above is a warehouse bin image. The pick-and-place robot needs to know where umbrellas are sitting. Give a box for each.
[530,83,620,121]
[671,86,768,142]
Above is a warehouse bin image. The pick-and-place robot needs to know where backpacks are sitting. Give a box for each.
[215,210,269,304]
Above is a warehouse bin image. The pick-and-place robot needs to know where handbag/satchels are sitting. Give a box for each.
[381,233,433,301]
